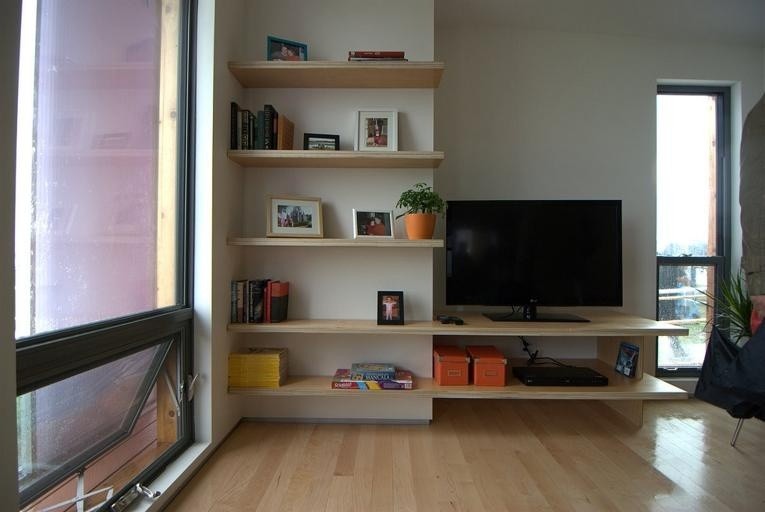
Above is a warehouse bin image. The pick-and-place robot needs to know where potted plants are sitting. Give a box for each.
[393,176,442,242]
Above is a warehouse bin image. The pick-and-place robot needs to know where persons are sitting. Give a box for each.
[273,46,300,61]
[366,216,385,235]
[383,297,397,320]
[278,208,293,227]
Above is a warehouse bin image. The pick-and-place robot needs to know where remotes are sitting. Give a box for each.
[448,317,464,325]
[437,315,450,324]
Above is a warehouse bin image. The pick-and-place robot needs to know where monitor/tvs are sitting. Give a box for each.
[446,200,622,322]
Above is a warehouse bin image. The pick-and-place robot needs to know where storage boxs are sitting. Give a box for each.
[464,344,508,387]
[435,346,470,386]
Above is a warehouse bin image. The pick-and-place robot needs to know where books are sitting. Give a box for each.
[230,101,294,150]
[348,51,407,62]
[330,362,413,390]
[227,279,289,387]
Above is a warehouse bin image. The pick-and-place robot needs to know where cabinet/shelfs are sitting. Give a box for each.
[434,312,690,427]
[226,62,444,422]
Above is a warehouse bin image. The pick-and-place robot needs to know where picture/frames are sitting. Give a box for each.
[267,34,308,62]
[354,106,401,155]
[302,131,340,150]
[376,289,405,327]
[264,190,324,240]
[351,206,396,240]
